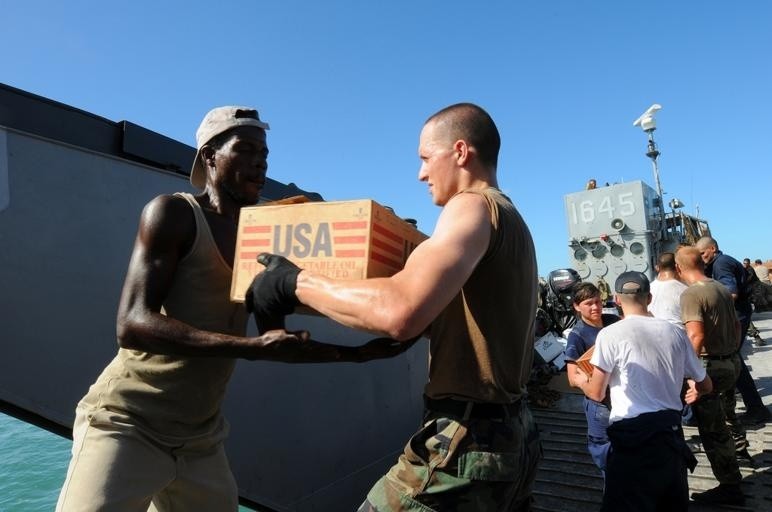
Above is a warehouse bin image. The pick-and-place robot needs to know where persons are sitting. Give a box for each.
[692,236,771,425]
[744,320,766,347]
[571,268,714,512]
[561,281,623,492]
[742,258,768,312]
[645,251,700,428]
[242,101,545,511]
[56,103,428,511]
[589,179,598,189]
[753,259,771,310]
[674,244,754,505]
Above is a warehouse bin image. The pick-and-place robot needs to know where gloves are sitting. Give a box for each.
[246,252,303,319]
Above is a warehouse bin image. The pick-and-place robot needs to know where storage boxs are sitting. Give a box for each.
[575,343,595,379]
[231,199,431,305]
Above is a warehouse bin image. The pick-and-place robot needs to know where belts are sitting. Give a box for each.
[423,394,528,419]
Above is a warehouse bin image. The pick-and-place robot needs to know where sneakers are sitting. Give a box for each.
[690,484,748,506]
[755,335,764,347]
[735,454,756,469]
[683,415,697,426]
[735,408,772,423]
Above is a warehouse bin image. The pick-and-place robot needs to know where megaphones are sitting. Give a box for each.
[609,217,626,231]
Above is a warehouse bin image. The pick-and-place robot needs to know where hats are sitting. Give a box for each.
[189,104,268,189]
[615,270,651,294]
